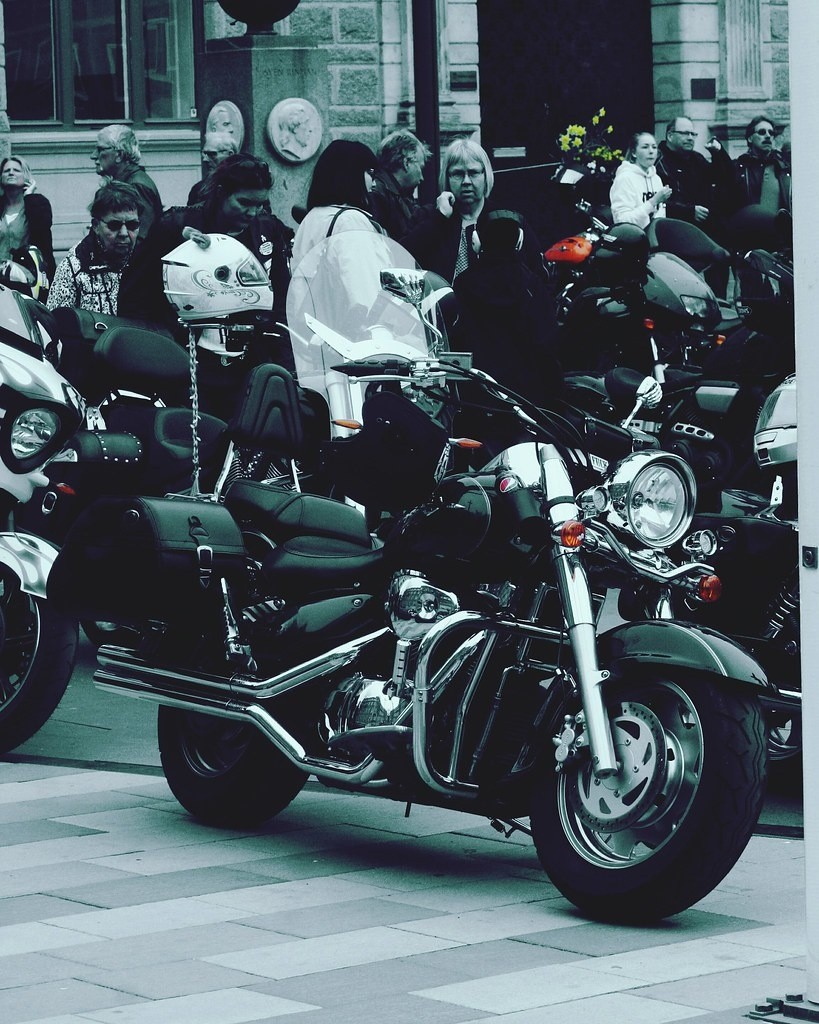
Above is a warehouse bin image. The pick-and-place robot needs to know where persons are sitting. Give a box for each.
[275,104,312,160]
[90,125,164,244]
[156,115,792,439]
[0,156,56,284]
[209,107,233,135]
[45,183,144,316]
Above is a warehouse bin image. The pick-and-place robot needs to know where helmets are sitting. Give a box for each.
[160,232,275,320]
[590,222,650,277]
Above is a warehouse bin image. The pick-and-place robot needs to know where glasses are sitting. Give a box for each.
[671,130,698,138]
[748,128,776,137]
[95,145,116,153]
[227,161,269,171]
[96,216,141,232]
[448,167,486,180]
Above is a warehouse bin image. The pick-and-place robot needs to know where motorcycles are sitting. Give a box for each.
[1,197,801,921]
